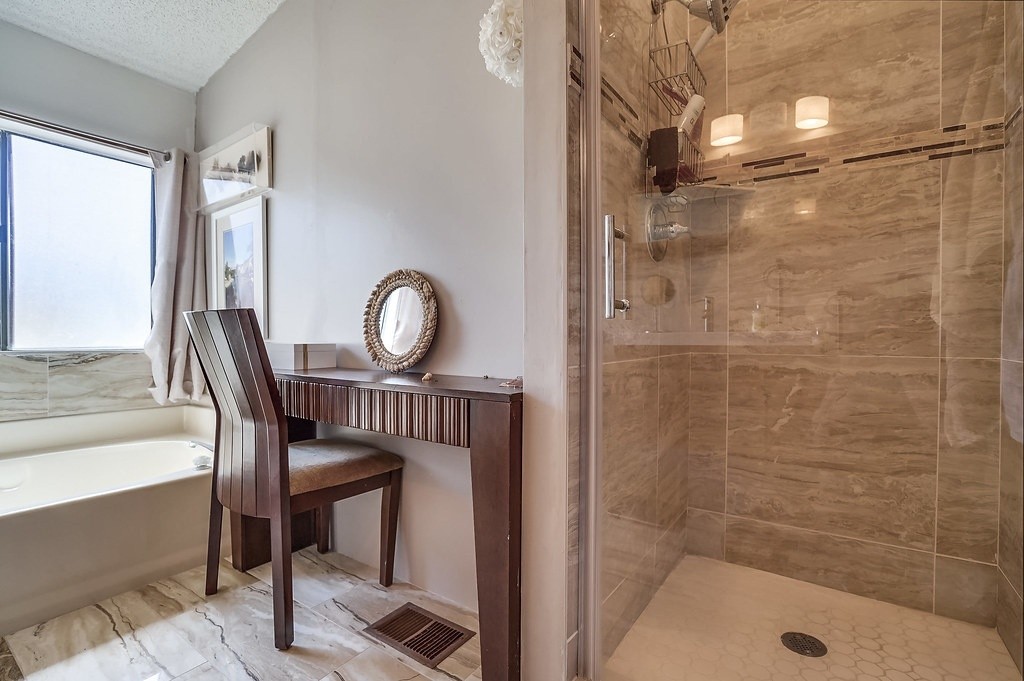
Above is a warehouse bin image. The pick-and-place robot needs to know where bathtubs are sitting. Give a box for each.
[0,438,217,638]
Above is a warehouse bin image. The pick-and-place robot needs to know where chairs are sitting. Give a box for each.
[184,307,405,648]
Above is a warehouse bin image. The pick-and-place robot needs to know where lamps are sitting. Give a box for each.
[795,97,830,129]
[710,114,743,145]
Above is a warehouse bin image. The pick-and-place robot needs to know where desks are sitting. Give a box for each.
[229,365,523,681]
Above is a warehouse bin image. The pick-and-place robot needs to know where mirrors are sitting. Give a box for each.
[364,266,436,371]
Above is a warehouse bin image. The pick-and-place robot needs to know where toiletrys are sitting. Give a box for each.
[752,299,766,332]
[675,94,705,153]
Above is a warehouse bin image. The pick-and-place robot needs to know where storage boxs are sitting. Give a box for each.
[267,340,337,371]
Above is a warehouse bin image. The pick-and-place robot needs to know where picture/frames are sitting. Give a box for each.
[212,195,268,338]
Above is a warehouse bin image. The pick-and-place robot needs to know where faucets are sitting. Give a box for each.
[191,455,212,470]
[702,296,713,332]
